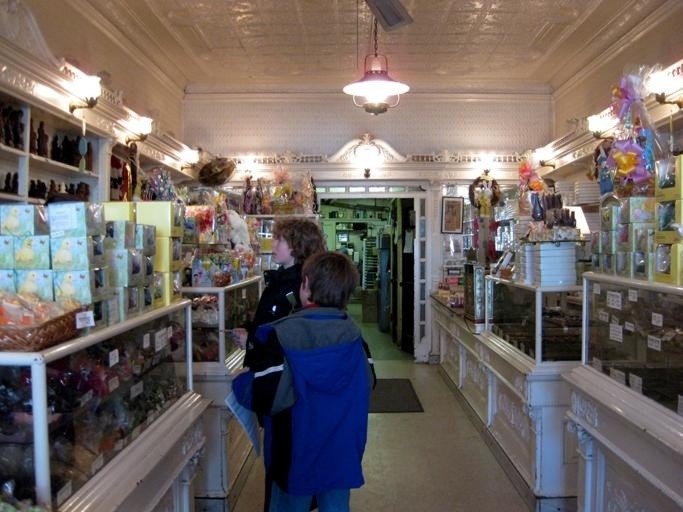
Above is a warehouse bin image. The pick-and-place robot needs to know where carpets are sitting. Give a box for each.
[367,378,423,414]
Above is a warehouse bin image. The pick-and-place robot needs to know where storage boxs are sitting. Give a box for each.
[575,153,681,289]
[361,289,378,325]
[1,198,188,335]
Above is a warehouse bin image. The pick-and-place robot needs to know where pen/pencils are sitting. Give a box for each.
[219,330,232,333]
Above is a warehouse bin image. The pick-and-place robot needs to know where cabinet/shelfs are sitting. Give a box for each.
[429,291,490,439]
[480,275,586,512]
[560,266,682,512]
[235,213,320,300]
[179,271,266,511]
[2,290,211,512]
[460,218,474,253]
[0,74,112,206]
[109,132,192,203]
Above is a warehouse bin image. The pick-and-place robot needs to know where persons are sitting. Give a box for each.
[222,220,377,512]
[231,249,374,511]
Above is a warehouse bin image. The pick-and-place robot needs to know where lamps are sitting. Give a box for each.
[179,148,200,171]
[535,149,555,170]
[341,0,411,117]
[66,73,103,112]
[644,73,682,111]
[123,117,153,146]
[585,115,617,141]
[481,157,492,178]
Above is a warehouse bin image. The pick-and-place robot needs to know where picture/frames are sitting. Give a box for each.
[439,195,464,235]
